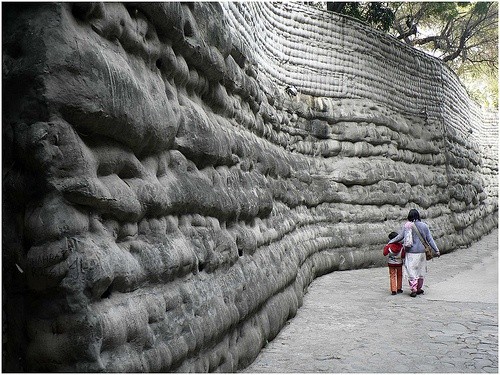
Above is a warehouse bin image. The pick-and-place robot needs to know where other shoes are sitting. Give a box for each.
[397,289,403,293]
[417,290,424,295]
[410,293,416,297]
[392,291,396,294]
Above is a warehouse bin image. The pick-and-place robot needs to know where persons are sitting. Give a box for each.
[383,232,406,296]
[385,209,441,297]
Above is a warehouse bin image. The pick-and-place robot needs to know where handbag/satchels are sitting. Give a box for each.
[425,243,433,260]
